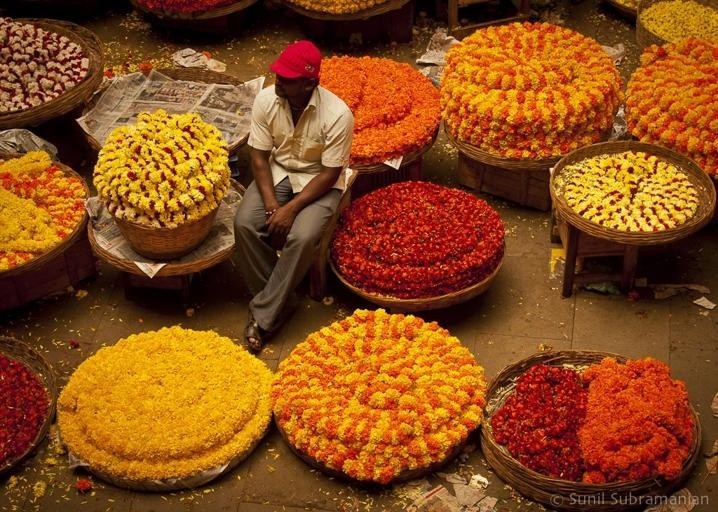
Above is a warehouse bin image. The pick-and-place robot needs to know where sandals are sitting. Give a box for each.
[244,300,297,351]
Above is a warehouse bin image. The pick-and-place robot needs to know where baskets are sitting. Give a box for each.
[281,1,718,312]
[0,336,703,511]
[1,0,253,279]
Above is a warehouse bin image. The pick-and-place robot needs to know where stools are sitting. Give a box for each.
[273,171,357,299]
[551,208,637,298]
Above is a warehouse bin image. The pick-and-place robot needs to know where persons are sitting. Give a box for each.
[234,40,354,353]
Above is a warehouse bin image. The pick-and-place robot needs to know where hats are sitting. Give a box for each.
[270,40,321,78]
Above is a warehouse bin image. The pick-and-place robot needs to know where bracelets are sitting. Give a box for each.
[264,208,276,216]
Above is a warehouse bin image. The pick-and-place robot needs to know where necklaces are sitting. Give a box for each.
[292,106,304,111]
